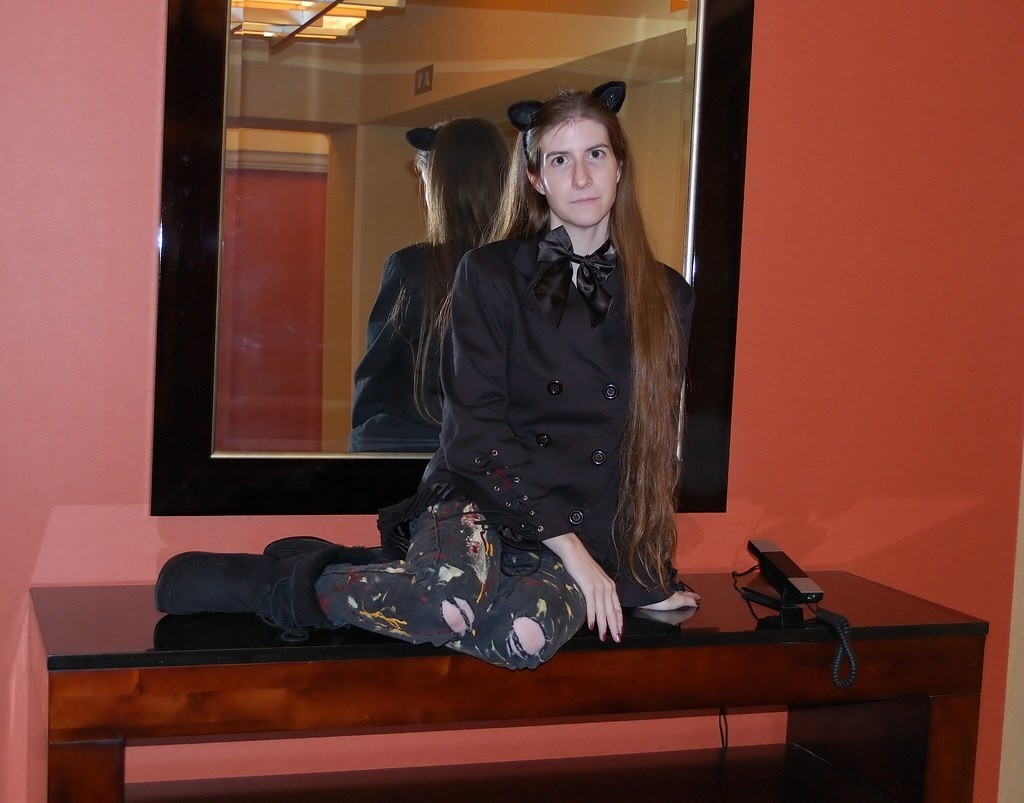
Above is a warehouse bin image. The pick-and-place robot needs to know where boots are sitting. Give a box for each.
[263,535,341,556]
[154,547,371,631]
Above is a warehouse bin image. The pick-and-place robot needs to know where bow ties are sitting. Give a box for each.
[532,225,617,329]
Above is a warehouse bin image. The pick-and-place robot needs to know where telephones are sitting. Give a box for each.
[744,538,824,611]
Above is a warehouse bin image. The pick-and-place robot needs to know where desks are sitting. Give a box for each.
[25,570,990,803]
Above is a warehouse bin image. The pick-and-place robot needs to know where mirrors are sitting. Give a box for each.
[147,0,753,512]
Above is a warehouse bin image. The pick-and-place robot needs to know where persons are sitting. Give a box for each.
[149,81,700,672]
[341,110,512,460]
